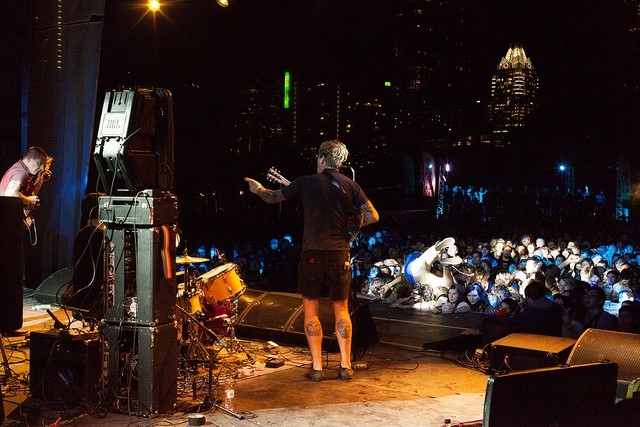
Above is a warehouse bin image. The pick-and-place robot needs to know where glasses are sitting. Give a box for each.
[316,154,320,157]
[468,282,481,287]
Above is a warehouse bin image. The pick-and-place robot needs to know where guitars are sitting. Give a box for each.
[266,167,365,245]
[18,157,54,229]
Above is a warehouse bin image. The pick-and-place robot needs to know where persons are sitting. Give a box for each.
[73,205,107,318]
[178,183,640,337]
[244,141,379,381]
[0,146,52,298]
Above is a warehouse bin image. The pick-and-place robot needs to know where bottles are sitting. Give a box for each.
[353,363,369,370]
[224,379,235,412]
[31,304,51,311]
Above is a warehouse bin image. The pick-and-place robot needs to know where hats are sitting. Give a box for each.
[481,243,491,250]
[557,278,576,294]
[615,257,631,265]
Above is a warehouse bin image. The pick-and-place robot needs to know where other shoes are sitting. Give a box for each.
[338,365,354,380]
[2,329,28,335]
[305,364,324,382]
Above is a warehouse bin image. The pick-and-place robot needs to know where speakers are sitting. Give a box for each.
[234,288,379,353]
[0,86,178,419]
[483,327,640,427]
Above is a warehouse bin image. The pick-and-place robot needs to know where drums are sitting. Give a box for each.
[177,296,238,346]
[196,262,246,307]
[174,288,203,315]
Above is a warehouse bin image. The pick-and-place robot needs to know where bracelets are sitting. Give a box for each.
[255,186,264,195]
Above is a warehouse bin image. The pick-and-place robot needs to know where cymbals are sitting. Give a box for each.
[176,257,210,264]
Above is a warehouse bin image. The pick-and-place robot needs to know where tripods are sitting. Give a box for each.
[172,304,245,419]
